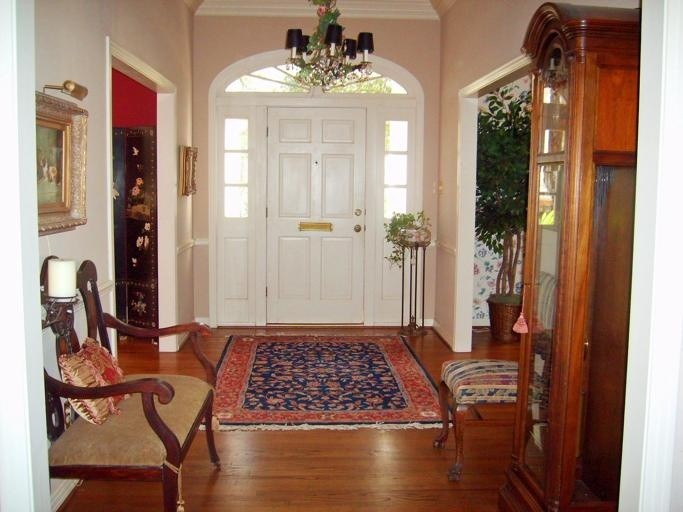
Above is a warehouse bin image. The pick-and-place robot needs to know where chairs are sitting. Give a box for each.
[36,251,227,510]
[433,269,563,481]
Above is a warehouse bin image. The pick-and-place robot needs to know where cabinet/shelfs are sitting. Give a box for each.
[396,241,431,337]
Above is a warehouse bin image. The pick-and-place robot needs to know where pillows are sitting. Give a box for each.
[56,334,130,428]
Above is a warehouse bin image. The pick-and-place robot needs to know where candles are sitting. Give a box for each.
[44,254,78,304]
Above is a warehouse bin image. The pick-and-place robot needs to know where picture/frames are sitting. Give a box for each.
[177,145,198,198]
[33,92,87,237]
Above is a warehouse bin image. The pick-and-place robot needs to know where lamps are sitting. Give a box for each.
[279,0,376,86]
[42,77,89,102]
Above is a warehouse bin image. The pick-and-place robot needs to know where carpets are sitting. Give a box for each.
[196,332,458,432]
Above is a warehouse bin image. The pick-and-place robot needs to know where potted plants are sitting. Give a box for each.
[384,210,435,266]
[473,81,536,345]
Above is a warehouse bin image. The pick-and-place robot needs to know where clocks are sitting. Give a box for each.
[498,0,643,510]
